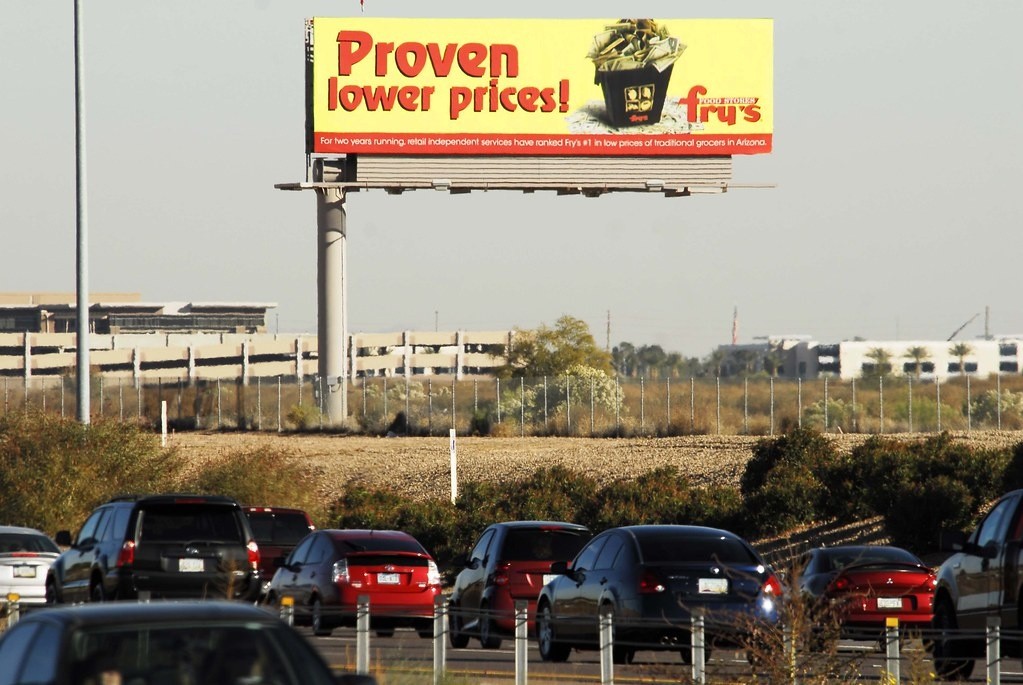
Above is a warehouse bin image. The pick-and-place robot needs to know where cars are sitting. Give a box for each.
[0,602,382,685]
[448,519,591,650]
[921,488,1023,681]
[258,528,442,639]
[242,505,317,585]
[0,525,62,618]
[775,544,936,653]
[534,525,786,666]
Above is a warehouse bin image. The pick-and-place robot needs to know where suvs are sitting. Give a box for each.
[44,491,264,606]
